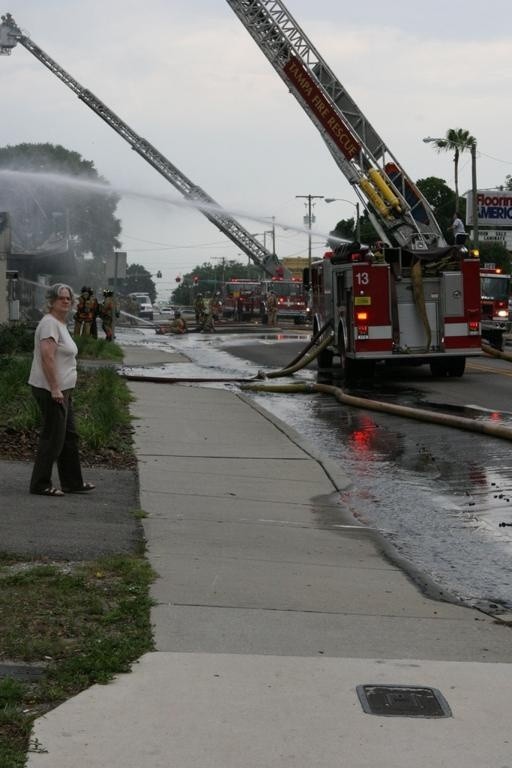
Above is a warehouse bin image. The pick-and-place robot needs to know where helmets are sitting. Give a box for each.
[102,290,115,299]
[81,286,96,296]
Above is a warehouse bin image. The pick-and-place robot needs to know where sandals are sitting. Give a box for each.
[30,487,65,496]
[62,483,96,491]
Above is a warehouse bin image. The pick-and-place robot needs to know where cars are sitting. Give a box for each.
[159,307,175,315]
[180,310,196,321]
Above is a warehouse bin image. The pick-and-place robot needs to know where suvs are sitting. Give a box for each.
[130,296,153,320]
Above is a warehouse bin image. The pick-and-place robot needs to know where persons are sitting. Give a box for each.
[447,212,469,253]
[27,284,96,497]
[194,290,223,334]
[73,286,141,342]
[266,291,278,328]
[155,310,185,334]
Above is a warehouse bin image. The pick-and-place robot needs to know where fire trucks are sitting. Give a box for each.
[224,0,484,381]
[480,268,512,352]
[1,13,309,325]
[219,280,262,317]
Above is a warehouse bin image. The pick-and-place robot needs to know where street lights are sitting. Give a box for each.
[423,137,478,259]
[324,197,360,244]
